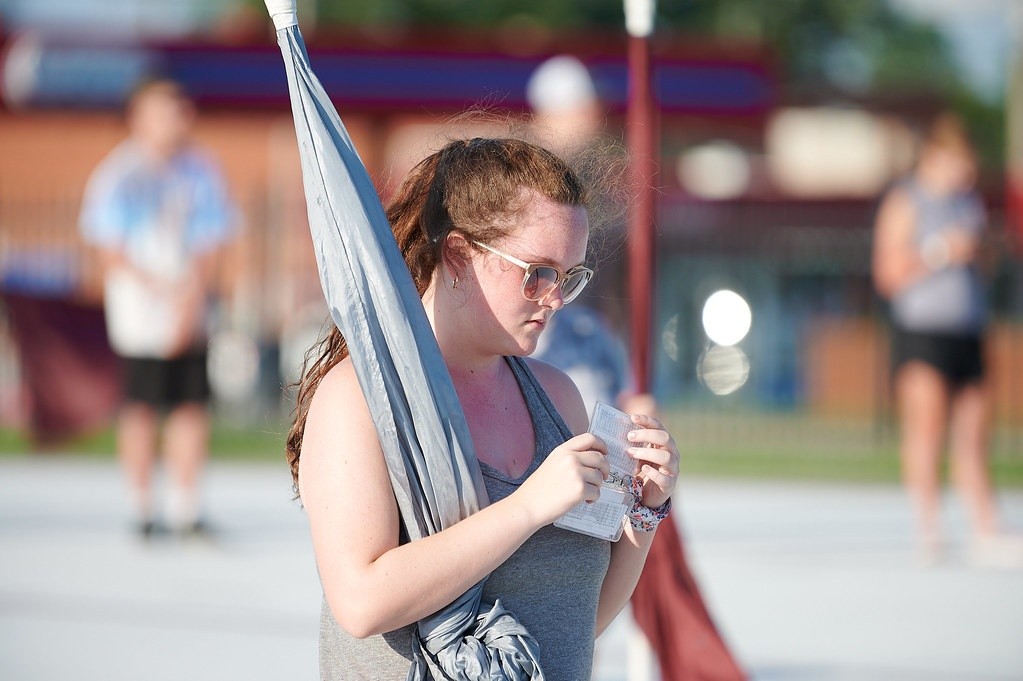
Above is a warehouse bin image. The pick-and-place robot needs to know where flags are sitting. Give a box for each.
[279,23,544,681]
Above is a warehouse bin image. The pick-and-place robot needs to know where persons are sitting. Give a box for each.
[80,85,242,540]
[285,138,680,681]
[873,116,994,539]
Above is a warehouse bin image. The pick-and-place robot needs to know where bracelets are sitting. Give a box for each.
[629,480,671,533]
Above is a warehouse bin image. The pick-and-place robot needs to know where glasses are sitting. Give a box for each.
[432,231,594,304]
